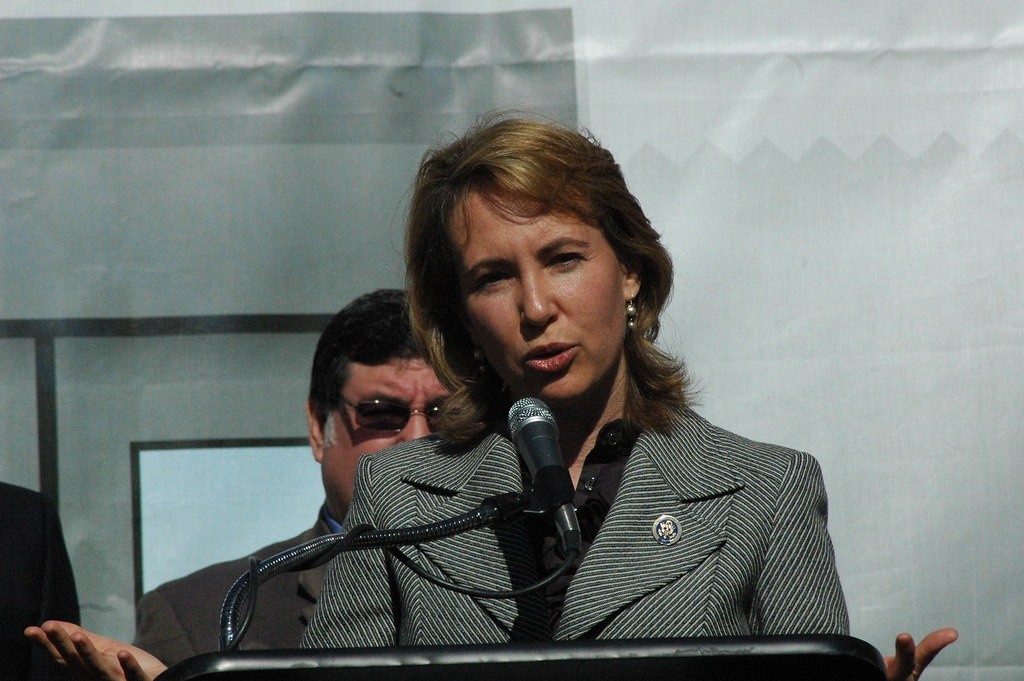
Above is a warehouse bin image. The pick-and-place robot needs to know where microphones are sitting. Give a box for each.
[509,397,581,554]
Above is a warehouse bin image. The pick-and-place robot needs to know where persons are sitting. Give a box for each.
[22,117,958,681]
[0,286,450,681]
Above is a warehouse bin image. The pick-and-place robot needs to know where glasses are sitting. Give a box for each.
[333,392,462,431]
